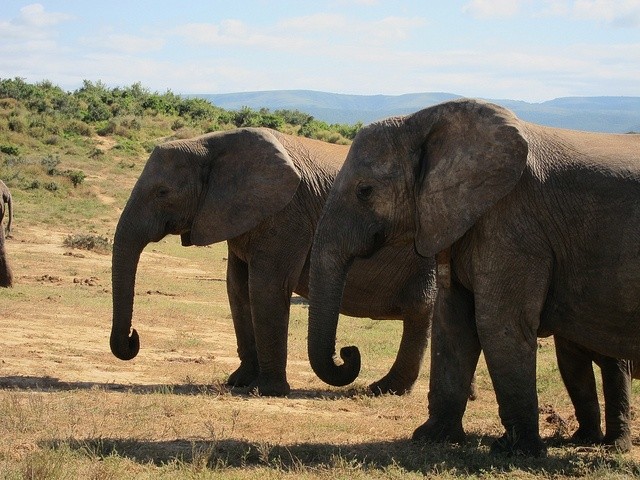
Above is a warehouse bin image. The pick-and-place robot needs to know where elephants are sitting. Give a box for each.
[0,178,15,289]
[306,95,639,465]
[106,125,480,403]
